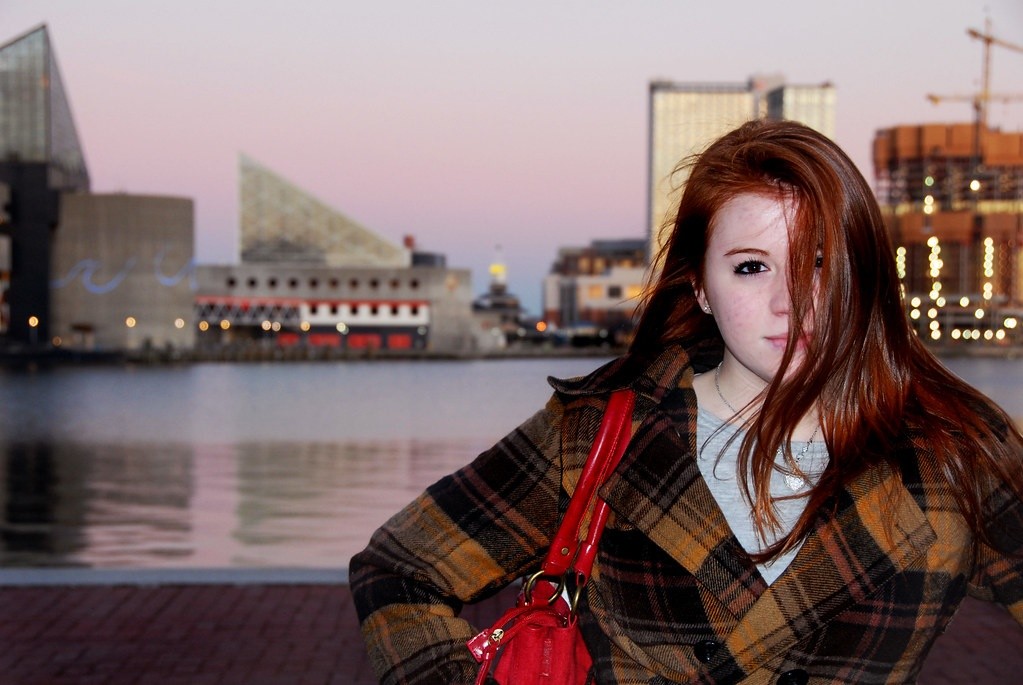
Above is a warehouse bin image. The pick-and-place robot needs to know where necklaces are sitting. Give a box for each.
[715,361,827,495]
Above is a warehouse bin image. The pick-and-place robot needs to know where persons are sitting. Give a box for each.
[349,120,1023,685]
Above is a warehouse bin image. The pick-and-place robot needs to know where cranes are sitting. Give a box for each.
[923,2,1023,160]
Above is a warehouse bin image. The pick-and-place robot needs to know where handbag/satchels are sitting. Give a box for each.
[465,390,635,685]
